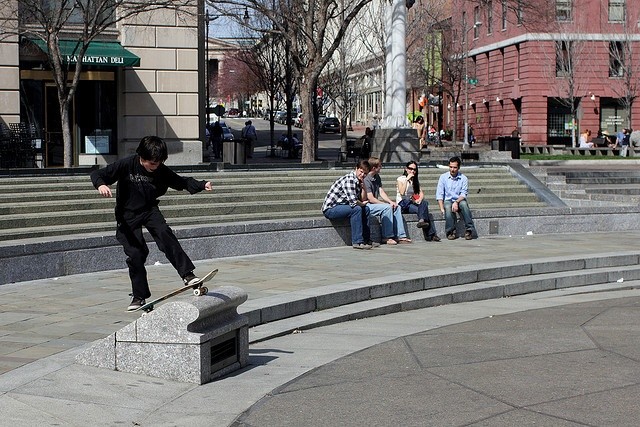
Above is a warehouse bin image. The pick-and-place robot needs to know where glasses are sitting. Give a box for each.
[378,165,382,167]
[407,167,417,171]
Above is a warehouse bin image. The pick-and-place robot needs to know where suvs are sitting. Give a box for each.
[294,112,303,127]
[225,108,239,116]
[279,111,297,125]
[319,117,340,133]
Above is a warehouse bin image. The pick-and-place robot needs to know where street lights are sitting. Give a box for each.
[204,6,250,130]
[463,21,482,150]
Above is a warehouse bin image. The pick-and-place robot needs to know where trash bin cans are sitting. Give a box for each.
[223,137,247,165]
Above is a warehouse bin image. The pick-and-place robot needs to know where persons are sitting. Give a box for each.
[436,157,476,240]
[283,134,289,148]
[360,127,375,160]
[412,116,425,150]
[241,122,248,138]
[362,157,412,245]
[206,125,211,149]
[322,161,381,250]
[578,127,640,156]
[243,120,257,158]
[207,121,224,161]
[291,133,303,144]
[90,136,214,312]
[395,161,442,242]
[277,133,286,146]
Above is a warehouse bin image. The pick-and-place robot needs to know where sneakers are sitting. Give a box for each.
[127,297,146,311]
[417,221,429,228]
[464,230,474,240]
[370,242,381,248]
[382,238,398,245]
[353,243,372,250]
[426,234,441,241]
[395,237,412,244]
[448,229,459,240]
[183,270,201,286]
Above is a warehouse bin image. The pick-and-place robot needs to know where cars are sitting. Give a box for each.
[211,121,226,127]
[221,126,234,141]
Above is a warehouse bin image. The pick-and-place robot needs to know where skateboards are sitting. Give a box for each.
[124,269,218,317]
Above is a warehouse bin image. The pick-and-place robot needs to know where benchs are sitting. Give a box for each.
[266,144,288,157]
[566,147,630,156]
[337,137,362,161]
[521,144,565,155]
